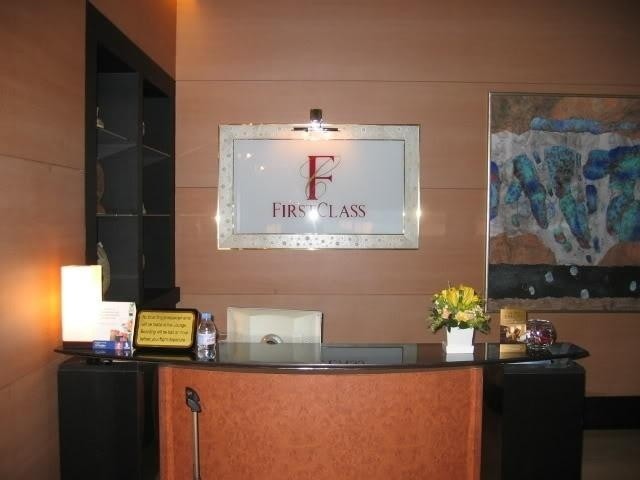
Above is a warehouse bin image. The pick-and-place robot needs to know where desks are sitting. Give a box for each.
[51,340,588,480]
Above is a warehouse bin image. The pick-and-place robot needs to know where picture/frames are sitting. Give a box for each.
[484,83,640,313]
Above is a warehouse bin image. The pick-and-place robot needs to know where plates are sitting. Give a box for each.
[442,352,474,363]
[442,341,475,354]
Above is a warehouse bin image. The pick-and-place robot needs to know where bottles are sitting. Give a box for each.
[197,313,216,362]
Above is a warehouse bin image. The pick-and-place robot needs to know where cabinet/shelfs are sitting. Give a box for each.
[84,8,180,311]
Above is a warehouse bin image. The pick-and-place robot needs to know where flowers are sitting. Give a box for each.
[426,283,492,334]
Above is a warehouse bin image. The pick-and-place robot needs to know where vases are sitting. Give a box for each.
[442,327,476,354]
